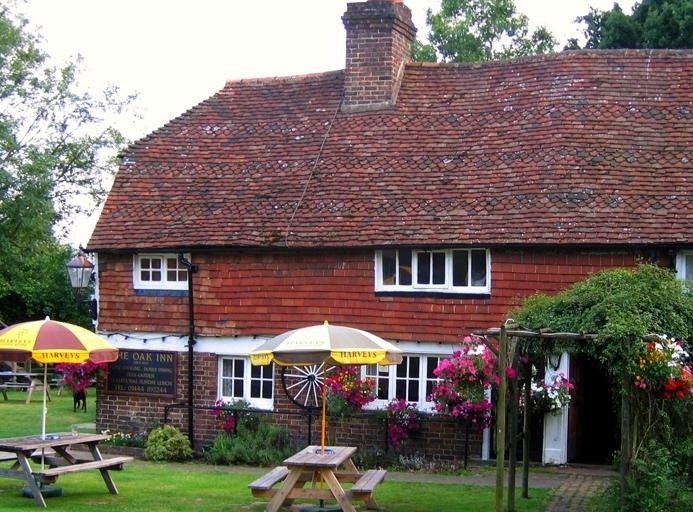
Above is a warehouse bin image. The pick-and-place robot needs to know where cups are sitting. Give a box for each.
[70,424,80,439]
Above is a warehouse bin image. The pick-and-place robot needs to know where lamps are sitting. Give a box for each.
[65,242,98,320]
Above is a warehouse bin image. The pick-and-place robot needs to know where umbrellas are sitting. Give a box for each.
[0,315,118,488]
[249,319,403,508]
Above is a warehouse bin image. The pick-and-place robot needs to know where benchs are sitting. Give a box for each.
[0,451,135,509]
[246,466,388,511]
[0,382,61,403]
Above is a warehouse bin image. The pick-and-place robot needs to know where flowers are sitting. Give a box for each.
[384,398,427,455]
[53,359,101,392]
[319,363,381,418]
[410,329,577,435]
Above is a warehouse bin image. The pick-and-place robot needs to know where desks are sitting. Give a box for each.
[0,372,53,403]
[0,430,119,508]
[263,444,359,511]
